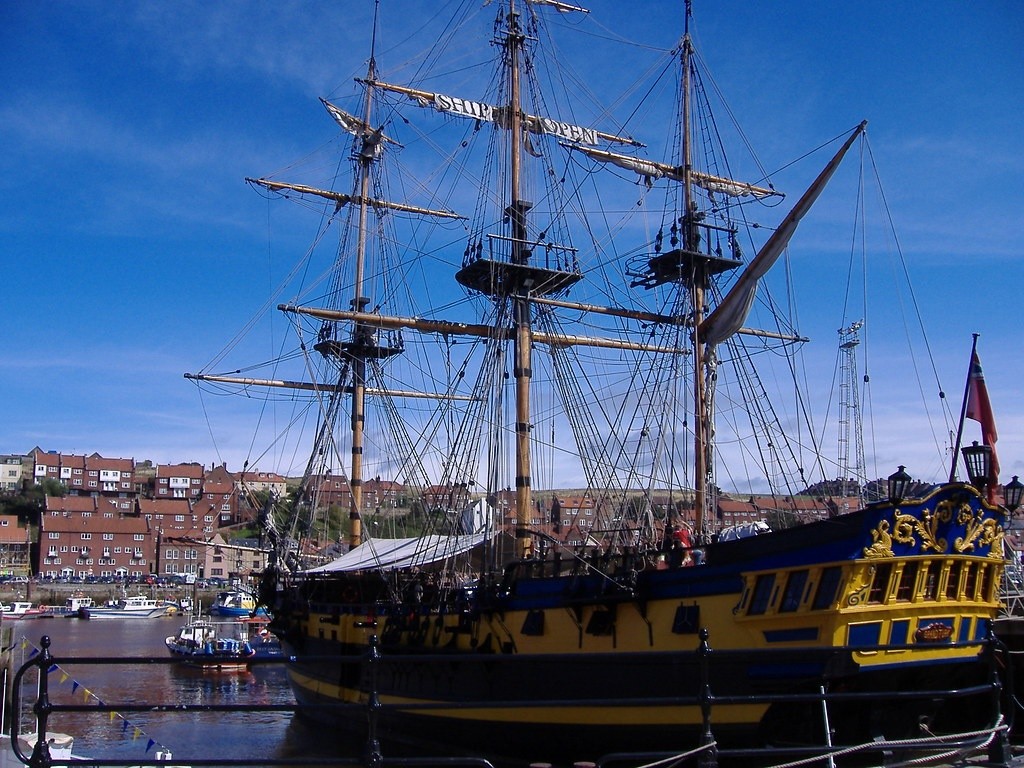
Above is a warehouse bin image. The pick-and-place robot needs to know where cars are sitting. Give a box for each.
[0,574,28,585]
[31,573,157,585]
[169,574,228,588]
[154,577,168,588]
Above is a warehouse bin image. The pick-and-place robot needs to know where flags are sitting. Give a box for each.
[968,350,1001,506]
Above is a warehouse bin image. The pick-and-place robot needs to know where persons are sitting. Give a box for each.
[673,520,702,565]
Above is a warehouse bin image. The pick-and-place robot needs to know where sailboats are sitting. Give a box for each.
[165,0,1024,768]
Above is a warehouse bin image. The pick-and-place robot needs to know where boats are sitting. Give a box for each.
[1,595,92,619]
[83,596,171,620]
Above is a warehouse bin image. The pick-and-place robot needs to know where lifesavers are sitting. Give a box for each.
[256,625,271,639]
[39,604,46,612]
